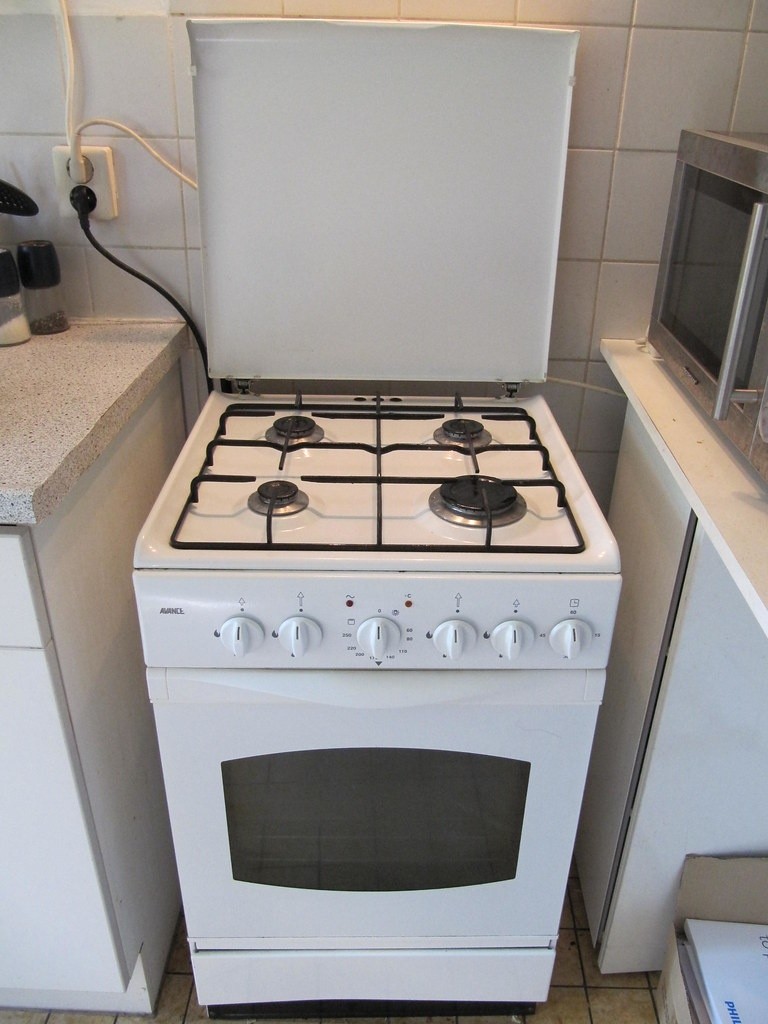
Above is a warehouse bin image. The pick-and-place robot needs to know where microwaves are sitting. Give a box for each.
[647,127,768,482]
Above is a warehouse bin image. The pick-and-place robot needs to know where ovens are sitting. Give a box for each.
[132,568,624,1018]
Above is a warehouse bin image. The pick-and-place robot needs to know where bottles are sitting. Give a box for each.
[0,247,32,346]
[18,239,70,335]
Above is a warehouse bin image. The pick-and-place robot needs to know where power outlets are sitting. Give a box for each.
[52,145,119,222]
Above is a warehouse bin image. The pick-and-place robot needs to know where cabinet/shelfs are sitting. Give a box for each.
[0,361,196,1016]
[575,392,768,975]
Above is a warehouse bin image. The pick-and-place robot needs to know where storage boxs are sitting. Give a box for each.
[650,850,768,1024]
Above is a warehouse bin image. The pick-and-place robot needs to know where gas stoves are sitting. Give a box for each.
[132,388,622,574]
